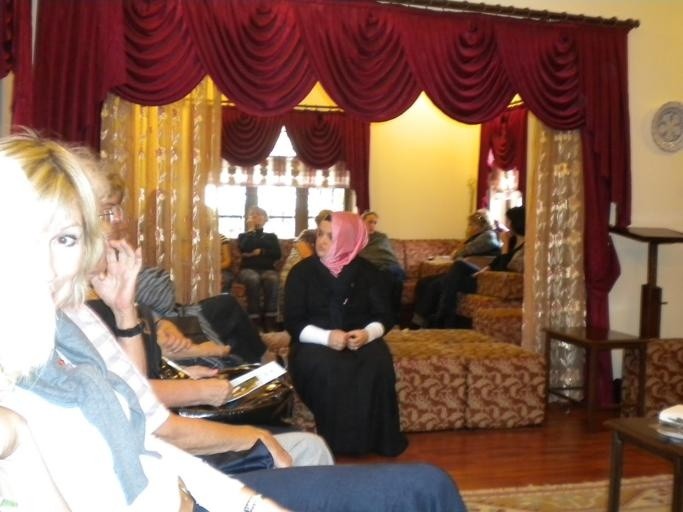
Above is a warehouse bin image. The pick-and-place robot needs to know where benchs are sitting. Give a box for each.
[261,330,549,434]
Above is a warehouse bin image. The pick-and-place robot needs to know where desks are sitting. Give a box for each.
[599,419,683,512]
[544,326,648,426]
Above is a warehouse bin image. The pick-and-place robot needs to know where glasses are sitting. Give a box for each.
[98,205,122,223]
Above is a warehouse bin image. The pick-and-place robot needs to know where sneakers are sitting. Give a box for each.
[250,316,279,332]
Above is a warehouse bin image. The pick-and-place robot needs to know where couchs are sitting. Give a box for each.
[228,236,523,333]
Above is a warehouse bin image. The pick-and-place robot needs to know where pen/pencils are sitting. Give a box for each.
[160,355,197,381]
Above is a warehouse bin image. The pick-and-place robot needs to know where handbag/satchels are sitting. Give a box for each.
[161,359,294,419]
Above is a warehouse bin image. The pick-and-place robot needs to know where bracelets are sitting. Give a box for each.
[115,320,147,339]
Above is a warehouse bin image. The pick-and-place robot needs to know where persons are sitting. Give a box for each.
[0,126,469,512]
[219,233,236,291]
[238,205,283,333]
[283,208,407,461]
[410,204,526,330]
[152,316,245,366]
[136,265,267,362]
[84,155,293,430]
[493,220,505,232]
[55,137,338,473]
[412,207,501,302]
[357,211,407,332]
[274,209,334,332]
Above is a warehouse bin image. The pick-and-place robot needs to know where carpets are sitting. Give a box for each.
[458,473,683,511]
